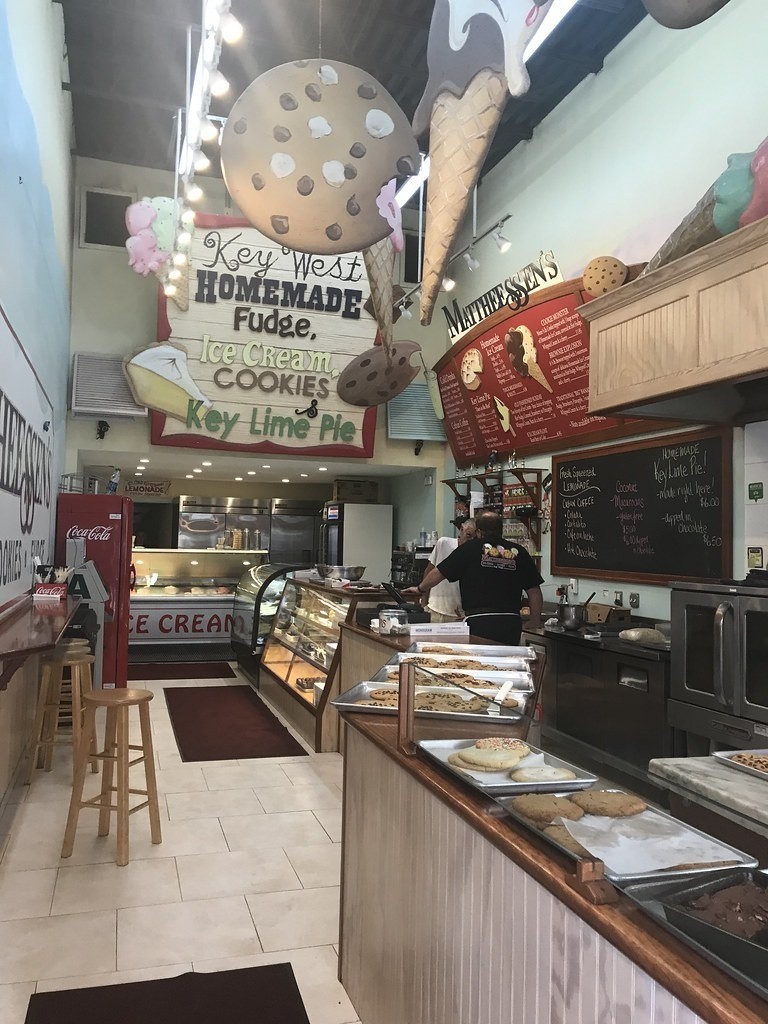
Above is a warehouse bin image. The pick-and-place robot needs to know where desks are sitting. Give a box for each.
[0,595,84,692]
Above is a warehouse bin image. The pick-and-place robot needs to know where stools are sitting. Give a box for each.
[59,688,162,866]
[23,638,100,785]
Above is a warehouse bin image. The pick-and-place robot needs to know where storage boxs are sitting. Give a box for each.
[333,479,378,503]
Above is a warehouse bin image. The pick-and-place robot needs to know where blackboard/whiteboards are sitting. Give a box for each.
[549,423,734,584]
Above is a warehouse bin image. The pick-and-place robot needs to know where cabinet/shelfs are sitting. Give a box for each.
[258,576,424,754]
[574,215,768,428]
[440,467,548,574]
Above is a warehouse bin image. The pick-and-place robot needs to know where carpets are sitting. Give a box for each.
[127,662,238,681]
[24,961,310,1024]
[163,684,309,763]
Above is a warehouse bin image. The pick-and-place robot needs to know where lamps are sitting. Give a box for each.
[190,143,210,172]
[441,278,456,292]
[174,224,191,245]
[165,258,182,280]
[162,276,178,297]
[398,305,412,320]
[462,252,481,274]
[177,197,197,223]
[181,174,204,202]
[170,249,186,265]
[202,61,229,98]
[196,108,217,141]
[492,232,512,253]
[216,4,244,44]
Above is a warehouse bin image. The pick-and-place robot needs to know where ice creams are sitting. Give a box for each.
[363,179,404,369]
[409,1,554,325]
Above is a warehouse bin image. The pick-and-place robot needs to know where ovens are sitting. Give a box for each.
[664,581,768,750]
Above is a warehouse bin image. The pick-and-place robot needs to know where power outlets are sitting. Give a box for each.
[629,593,639,609]
[569,578,578,594]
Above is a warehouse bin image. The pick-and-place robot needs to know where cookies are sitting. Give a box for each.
[511,789,738,871]
[447,735,576,783]
[355,646,518,713]
[730,753,768,774]
[582,257,627,297]
[218,59,423,257]
[337,340,423,408]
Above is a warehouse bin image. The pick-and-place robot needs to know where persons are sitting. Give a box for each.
[400,512,545,647]
[422,517,476,623]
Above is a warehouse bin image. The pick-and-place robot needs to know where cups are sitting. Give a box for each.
[426,531,438,547]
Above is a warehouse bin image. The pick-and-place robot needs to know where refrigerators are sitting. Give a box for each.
[57,493,136,690]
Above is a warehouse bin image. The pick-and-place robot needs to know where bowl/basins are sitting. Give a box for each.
[558,603,588,630]
[516,508,537,516]
[315,564,366,580]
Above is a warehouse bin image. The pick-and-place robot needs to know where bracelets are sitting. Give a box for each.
[418,586,426,593]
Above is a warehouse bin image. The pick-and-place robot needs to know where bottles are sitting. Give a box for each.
[380,609,409,635]
[224,528,260,550]
[502,523,536,556]
[392,558,415,583]
[420,527,426,546]
[474,481,536,518]
[395,541,412,552]
[107,468,121,494]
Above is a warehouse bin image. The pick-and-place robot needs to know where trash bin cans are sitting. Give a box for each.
[58,608,101,727]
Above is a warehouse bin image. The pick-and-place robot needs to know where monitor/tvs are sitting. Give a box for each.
[77,560,110,602]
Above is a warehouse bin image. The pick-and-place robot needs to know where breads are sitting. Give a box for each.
[619,627,666,643]
[676,882,768,950]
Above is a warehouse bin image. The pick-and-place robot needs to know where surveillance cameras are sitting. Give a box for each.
[99,421,110,432]
